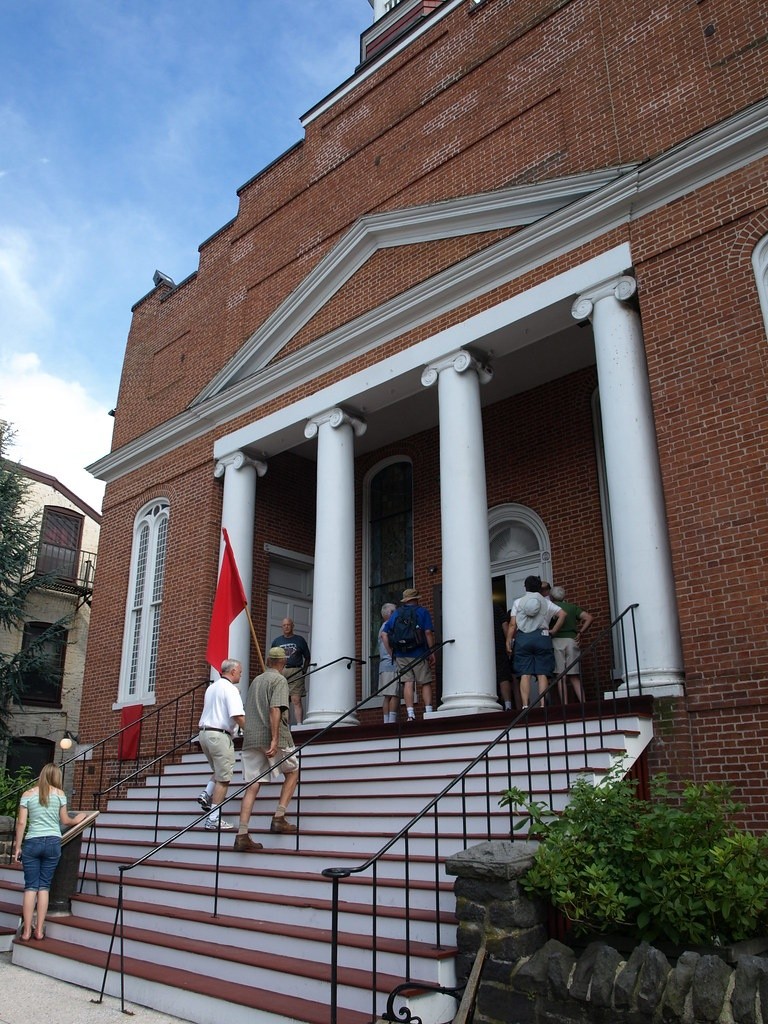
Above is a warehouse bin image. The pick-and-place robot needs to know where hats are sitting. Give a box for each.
[268,647,287,659]
[516,593,547,633]
[400,589,422,602]
[549,587,565,601]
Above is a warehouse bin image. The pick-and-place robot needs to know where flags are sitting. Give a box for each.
[205,531,247,674]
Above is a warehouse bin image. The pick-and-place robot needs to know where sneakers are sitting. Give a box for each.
[233,834,263,850]
[270,817,296,832]
[206,818,233,828]
[197,791,211,813]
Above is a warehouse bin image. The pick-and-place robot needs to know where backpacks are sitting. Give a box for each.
[392,605,420,653]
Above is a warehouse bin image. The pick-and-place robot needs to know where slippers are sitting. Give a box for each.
[34,924,45,940]
[19,925,33,942]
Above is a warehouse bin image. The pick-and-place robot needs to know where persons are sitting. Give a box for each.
[381,589,435,721]
[15,764,87,941]
[540,582,594,704]
[271,618,310,725]
[197,659,245,829]
[378,603,399,723]
[505,576,568,709]
[233,647,299,850]
[493,606,512,712]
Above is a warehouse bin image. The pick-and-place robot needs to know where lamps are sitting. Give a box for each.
[60,730,83,749]
[152,269,175,286]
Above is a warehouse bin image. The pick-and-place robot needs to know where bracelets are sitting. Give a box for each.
[578,631,581,635]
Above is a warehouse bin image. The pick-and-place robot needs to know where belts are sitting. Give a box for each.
[284,665,302,668]
[199,727,231,735]
